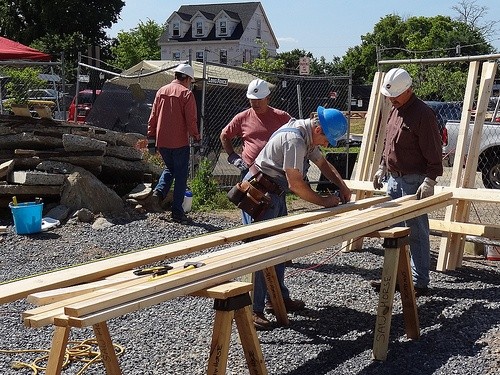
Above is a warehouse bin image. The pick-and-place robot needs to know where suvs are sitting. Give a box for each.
[282,78,341,118]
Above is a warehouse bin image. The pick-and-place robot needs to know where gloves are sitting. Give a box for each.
[372,164,388,190]
[416,177,438,199]
[228,152,248,171]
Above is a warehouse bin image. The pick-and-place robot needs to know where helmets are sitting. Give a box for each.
[174,64,196,82]
[317,105,347,146]
[380,68,412,98]
[246,79,270,99]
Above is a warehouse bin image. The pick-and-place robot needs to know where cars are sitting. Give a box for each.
[1,88,69,110]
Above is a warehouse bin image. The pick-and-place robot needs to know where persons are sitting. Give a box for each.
[370,69,444,292]
[147,63,200,223]
[240,106,352,330]
[220,78,292,181]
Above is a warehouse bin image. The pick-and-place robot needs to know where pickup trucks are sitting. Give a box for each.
[440,119,500,189]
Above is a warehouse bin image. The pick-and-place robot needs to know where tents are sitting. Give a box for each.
[1,35,62,121]
[85,59,275,152]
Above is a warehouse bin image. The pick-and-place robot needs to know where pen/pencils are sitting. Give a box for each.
[327,188,332,196]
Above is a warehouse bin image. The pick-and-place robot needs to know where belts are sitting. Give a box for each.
[249,162,285,196]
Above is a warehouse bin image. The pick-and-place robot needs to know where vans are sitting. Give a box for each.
[66,90,151,128]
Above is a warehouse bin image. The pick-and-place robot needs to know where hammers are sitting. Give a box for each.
[147,262,206,282]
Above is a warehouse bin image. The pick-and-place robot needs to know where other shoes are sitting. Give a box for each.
[252,312,271,328]
[152,191,163,212]
[414,287,429,297]
[370,279,400,289]
[172,212,190,222]
[265,298,305,313]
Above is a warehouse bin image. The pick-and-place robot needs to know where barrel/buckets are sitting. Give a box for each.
[9,201,44,235]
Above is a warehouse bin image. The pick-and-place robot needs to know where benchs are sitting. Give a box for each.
[243,222,419,361]
[45,271,268,375]
